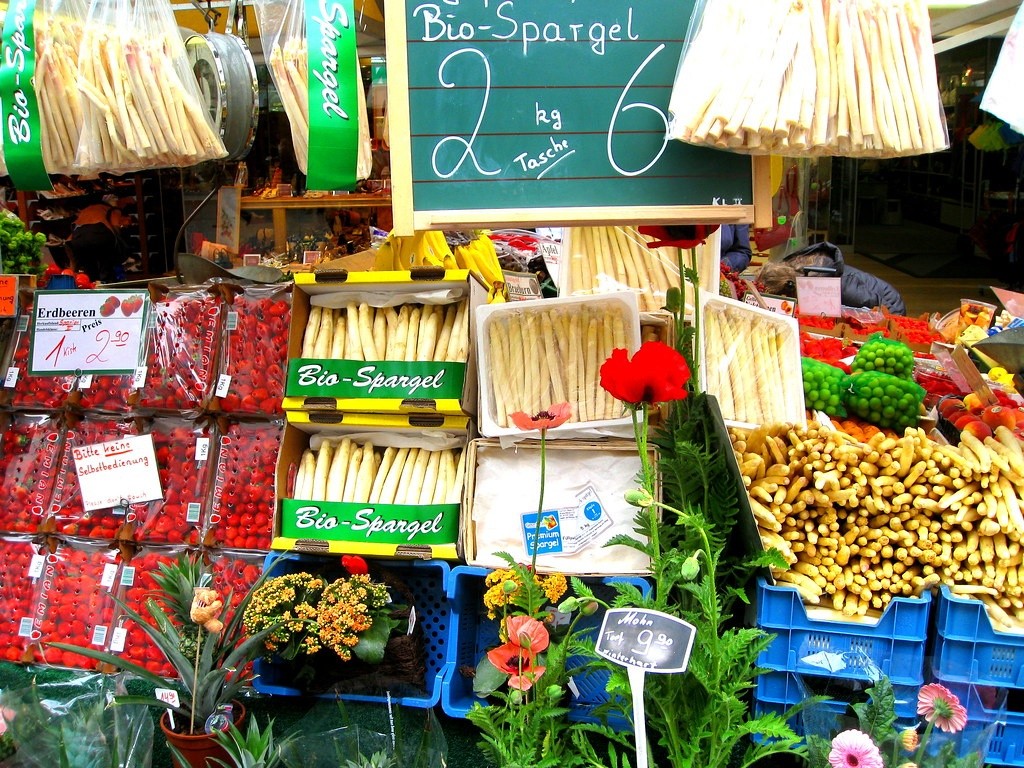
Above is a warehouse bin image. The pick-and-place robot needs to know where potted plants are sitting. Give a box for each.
[38,548,323,767]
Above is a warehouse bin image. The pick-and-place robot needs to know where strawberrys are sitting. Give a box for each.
[0,290,292,681]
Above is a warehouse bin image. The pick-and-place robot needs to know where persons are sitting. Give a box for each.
[754,241,907,318]
[720,223,753,276]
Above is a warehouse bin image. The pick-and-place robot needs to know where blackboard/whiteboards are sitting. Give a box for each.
[383,0,772,236]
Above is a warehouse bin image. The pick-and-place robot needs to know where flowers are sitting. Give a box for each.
[242,571,392,663]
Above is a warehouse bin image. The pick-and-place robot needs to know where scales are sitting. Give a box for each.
[172,18,284,286]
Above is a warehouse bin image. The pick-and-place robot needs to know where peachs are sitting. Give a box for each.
[939,394,1024,451]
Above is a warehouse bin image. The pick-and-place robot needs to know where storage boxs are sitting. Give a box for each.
[250,266,1024,768]
